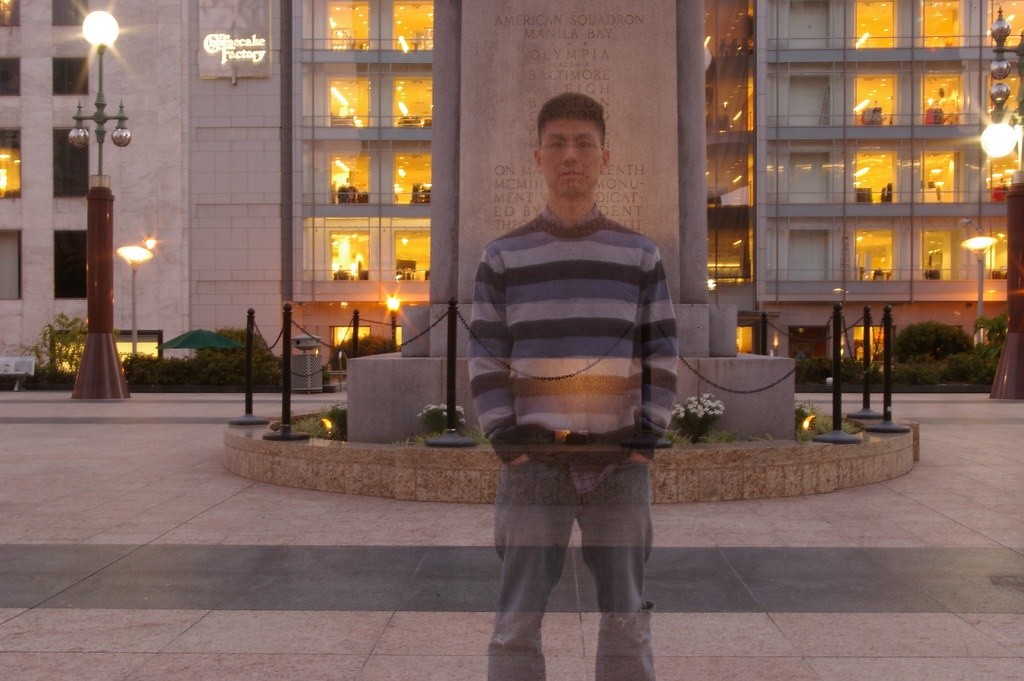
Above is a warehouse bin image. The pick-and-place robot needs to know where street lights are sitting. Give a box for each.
[66,11,132,400]
[978,3,1023,400]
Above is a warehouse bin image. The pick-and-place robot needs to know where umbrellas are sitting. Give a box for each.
[155,329,245,350]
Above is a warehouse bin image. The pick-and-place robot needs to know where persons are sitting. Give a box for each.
[466,92,679,681]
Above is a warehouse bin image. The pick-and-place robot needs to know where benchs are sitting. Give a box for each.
[0,356,35,392]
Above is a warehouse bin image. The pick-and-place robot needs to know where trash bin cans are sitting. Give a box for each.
[292,333,323,393]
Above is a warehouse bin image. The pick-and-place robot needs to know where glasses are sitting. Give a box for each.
[538,138,603,158]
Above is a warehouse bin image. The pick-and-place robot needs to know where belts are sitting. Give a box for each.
[516,429,636,446]
